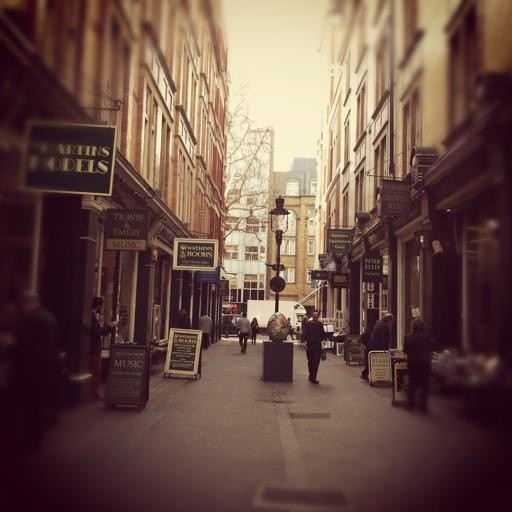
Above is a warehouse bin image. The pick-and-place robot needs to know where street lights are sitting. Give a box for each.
[265,194,291,313]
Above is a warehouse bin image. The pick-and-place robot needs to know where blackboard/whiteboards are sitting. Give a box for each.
[104,344,150,405]
[164,328,203,375]
[344,335,366,362]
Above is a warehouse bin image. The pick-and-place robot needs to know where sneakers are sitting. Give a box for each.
[308,376,319,383]
[361,371,369,381]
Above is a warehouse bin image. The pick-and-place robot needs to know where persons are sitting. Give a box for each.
[360,312,394,379]
[303,310,326,383]
[237,313,252,353]
[174,304,190,330]
[1,287,61,422]
[402,317,432,411]
[250,317,259,345]
[199,310,213,349]
[90,295,119,398]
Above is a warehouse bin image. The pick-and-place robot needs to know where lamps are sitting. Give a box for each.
[414,229,432,251]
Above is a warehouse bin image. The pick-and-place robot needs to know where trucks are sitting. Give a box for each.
[246,299,307,336]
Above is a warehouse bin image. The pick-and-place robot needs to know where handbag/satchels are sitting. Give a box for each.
[321,351,326,360]
